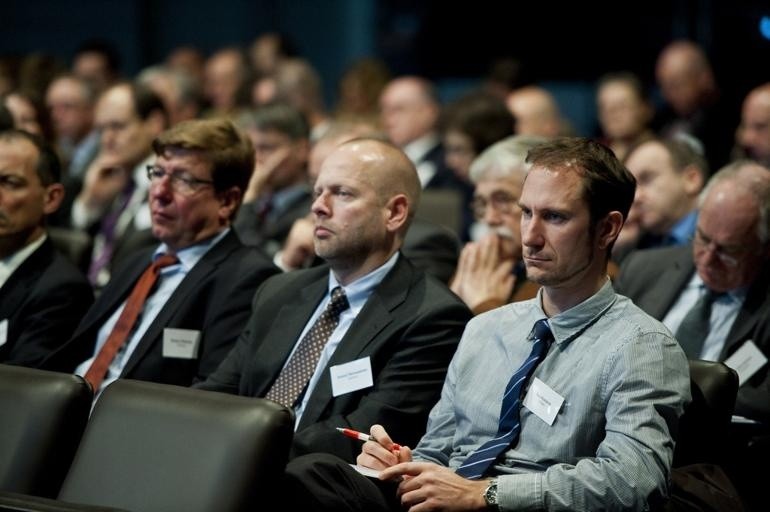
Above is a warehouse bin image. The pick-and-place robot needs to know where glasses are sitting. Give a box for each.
[685,231,760,268]
[471,193,517,216]
[146,164,212,188]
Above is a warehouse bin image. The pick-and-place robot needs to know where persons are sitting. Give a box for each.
[611,138,706,263]
[508,35,768,156]
[612,158,770,418]
[1,30,110,115]
[0,129,96,374]
[289,117,374,134]
[69,80,171,298]
[1,114,98,191]
[33,116,287,429]
[142,80,170,114]
[138,58,172,78]
[443,134,545,315]
[249,134,696,512]
[72,115,167,298]
[173,30,375,116]
[438,91,518,189]
[375,73,471,243]
[184,134,473,467]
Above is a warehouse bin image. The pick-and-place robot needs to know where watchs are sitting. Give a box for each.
[480,477,499,508]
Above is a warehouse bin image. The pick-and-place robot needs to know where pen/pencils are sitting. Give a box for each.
[335,427,400,452]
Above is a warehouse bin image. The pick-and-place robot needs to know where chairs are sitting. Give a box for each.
[0,377,297,512]
[0,361,94,511]
[413,189,464,241]
[684,358,739,464]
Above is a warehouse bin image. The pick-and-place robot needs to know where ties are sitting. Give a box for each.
[673,283,726,360]
[83,254,181,394]
[263,288,350,410]
[456,319,556,480]
